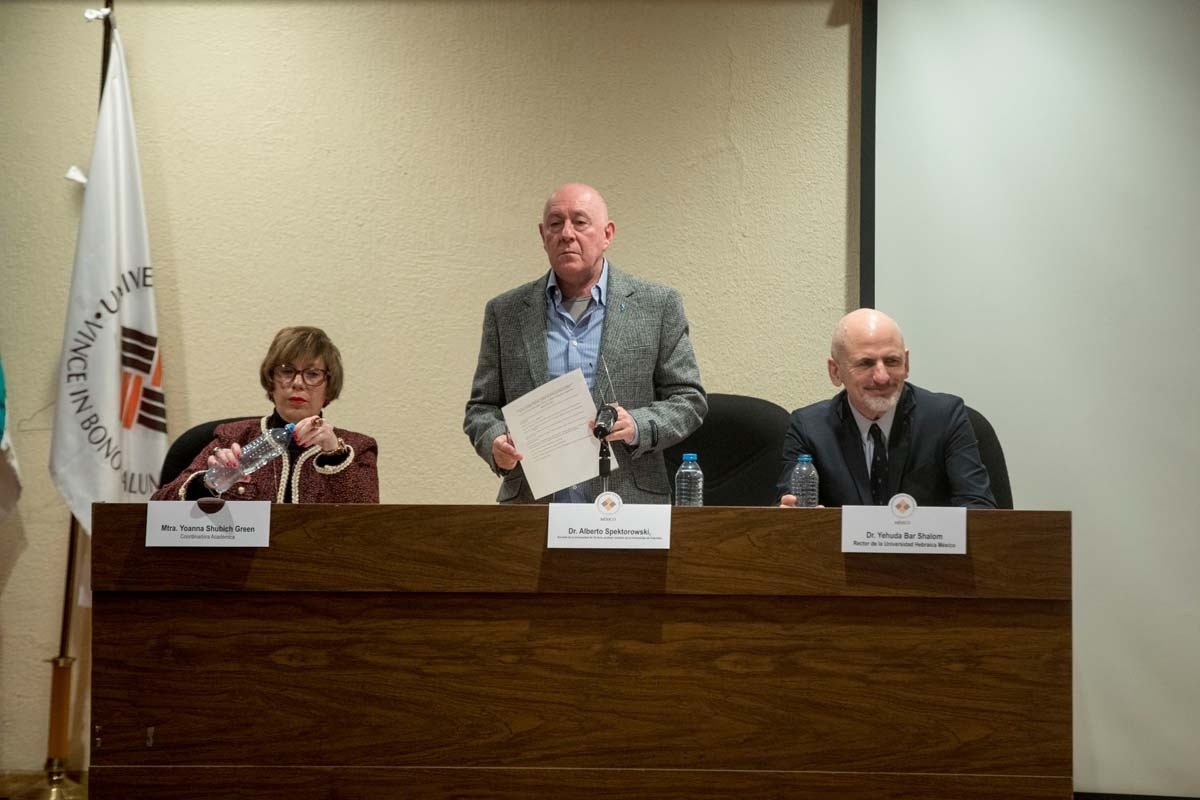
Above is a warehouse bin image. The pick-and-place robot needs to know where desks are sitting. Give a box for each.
[87,500,1075,800]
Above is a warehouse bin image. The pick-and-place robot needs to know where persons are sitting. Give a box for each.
[774,309,997,509]
[150,325,379,503]
[463,183,709,504]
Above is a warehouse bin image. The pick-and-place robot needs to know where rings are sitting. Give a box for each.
[312,417,323,428]
[212,447,222,453]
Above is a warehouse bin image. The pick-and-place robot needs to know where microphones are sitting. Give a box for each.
[592,405,617,439]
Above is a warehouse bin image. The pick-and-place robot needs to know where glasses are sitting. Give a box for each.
[271,365,327,386]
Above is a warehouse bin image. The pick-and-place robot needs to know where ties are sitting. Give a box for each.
[870,424,889,505]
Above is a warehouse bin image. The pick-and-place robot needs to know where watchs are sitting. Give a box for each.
[320,437,347,455]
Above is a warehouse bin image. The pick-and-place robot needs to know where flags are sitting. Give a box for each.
[51,12,171,608]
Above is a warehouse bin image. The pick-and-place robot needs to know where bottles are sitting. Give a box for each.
[792,455,819,507]
[204,423,296,493]
[675,454,703,507]
